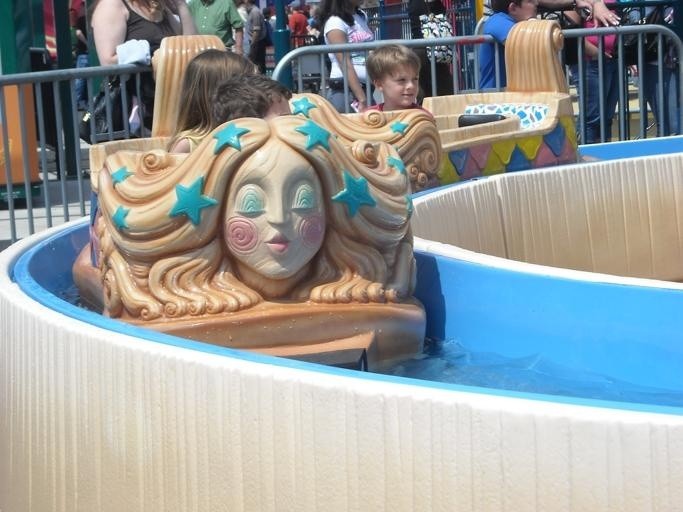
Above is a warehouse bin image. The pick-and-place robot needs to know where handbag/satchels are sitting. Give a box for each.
[328,78,344,90]
[541,13,583,65]
[78,74,132,141]
[420,13,454,64]
[612,6,667,66]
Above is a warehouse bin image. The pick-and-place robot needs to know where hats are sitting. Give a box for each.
[288,0,302,9]
[306,17,317,28]
